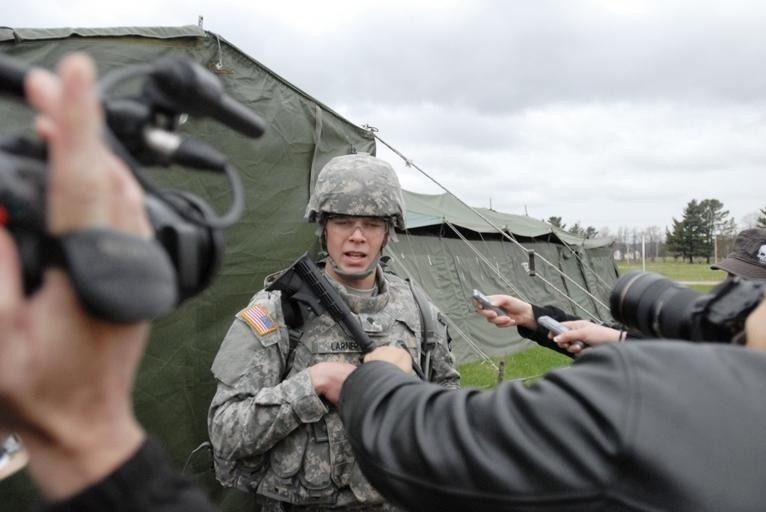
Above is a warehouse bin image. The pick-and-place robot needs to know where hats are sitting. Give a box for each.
[711,230,766,285]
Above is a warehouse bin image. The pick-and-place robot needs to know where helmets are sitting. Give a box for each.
[306,153,405,229]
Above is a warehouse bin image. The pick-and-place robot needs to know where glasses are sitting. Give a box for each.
[325,219,388,235]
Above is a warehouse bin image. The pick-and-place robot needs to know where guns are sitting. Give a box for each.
[266,251,377,363]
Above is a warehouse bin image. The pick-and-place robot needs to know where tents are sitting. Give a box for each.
[1,26,377,481]
[382,190,626,367]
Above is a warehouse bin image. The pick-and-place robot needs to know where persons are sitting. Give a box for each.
[466,229,766,355]
[1,53,214,512]
[338,292,766,509]
[207,152,463,509]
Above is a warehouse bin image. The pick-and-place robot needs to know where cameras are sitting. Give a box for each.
[610,270,766,343]
